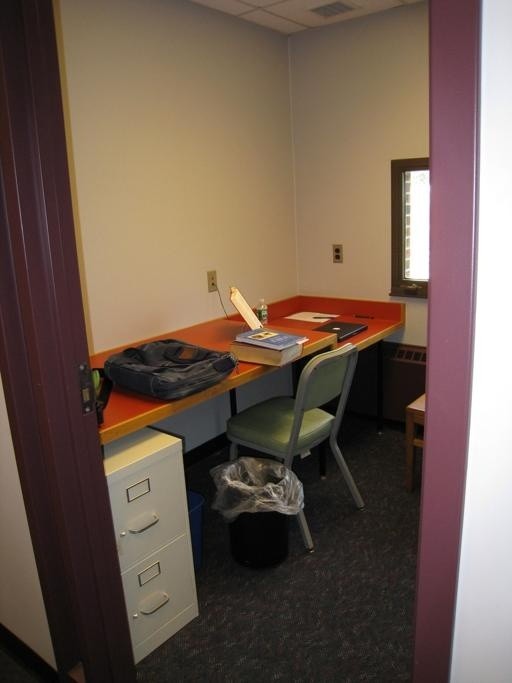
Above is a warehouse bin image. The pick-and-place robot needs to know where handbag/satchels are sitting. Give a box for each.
[104,338,238,401]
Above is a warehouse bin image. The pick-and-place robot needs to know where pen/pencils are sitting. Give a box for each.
[355,314,374,320]
[312,317,336,319]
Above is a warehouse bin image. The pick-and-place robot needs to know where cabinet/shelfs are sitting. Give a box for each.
[102,425,200,664]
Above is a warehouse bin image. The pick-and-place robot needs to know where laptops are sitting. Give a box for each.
[313,322,368,343]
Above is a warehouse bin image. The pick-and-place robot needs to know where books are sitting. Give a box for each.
[228,340,303,367]
[235,326,306,351]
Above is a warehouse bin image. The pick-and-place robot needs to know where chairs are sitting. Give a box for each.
[227,342,366,553]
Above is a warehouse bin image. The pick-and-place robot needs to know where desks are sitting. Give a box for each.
[87,296,405,665]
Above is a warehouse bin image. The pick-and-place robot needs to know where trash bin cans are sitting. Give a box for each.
[221,456,290,568]
[186,490,206,570]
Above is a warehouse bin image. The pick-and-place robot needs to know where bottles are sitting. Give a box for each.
[257,298,268,323]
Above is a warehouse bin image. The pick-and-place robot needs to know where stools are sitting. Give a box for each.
[406,391,425,493]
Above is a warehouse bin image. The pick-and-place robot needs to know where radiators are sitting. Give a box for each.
[348,341,426,423]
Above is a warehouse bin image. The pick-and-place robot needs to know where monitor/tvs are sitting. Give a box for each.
[388,157,430,298]
